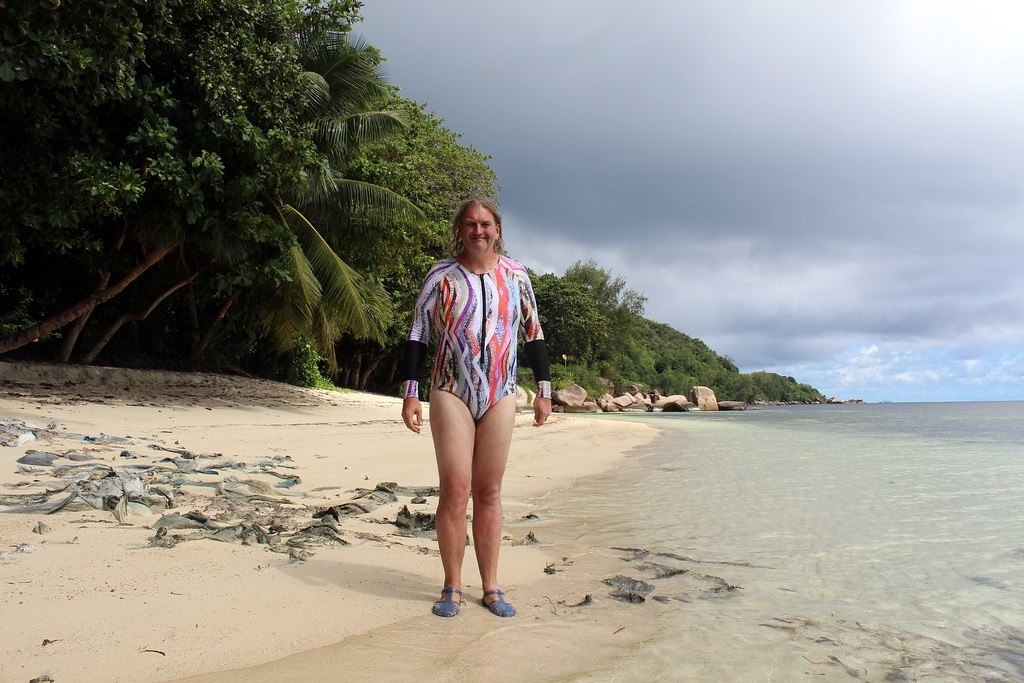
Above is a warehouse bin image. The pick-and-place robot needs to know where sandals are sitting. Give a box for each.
[433,588,462,617]
[481,590,517,617]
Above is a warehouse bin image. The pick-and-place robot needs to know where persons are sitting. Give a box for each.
[401,198,552,616]
